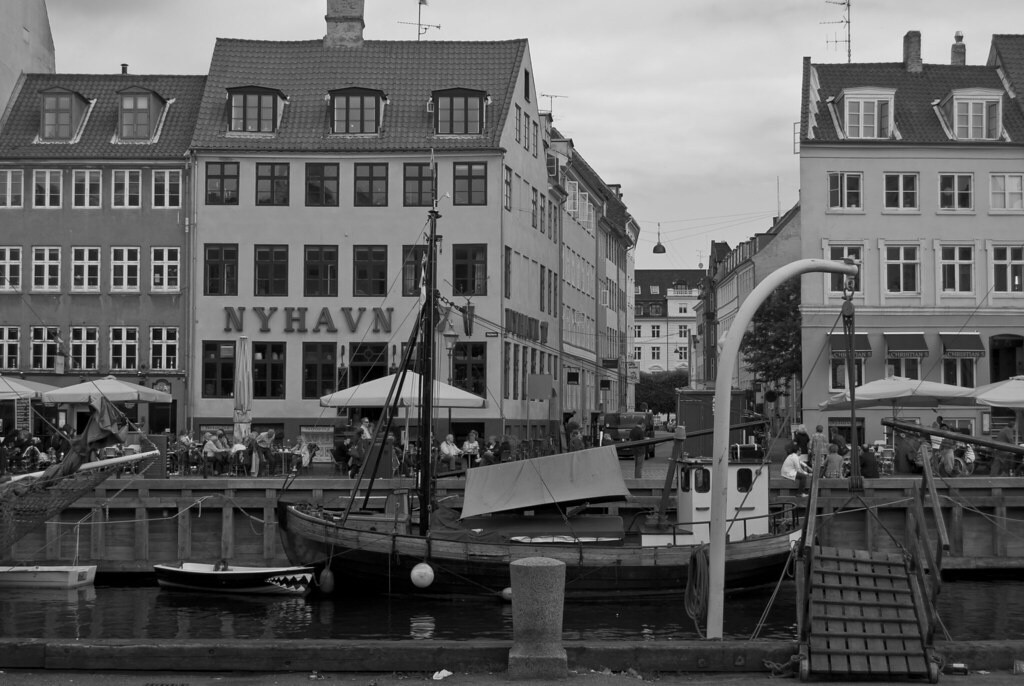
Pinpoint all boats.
[1,563,98,591]
[880,240,1024,577]
[1,274,161,560]
[153,554,315,598]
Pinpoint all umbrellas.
[975,375,1024,444]
[320,368,489,452]
[818,374,976,426]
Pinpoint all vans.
[601,411,656,460]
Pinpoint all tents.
[41,376,172,434]
[0,374,64,436]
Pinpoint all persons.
[0,414,1019,485]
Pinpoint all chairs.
[457,437,546,464]
[845,444,895,474]
[165,440,320,477]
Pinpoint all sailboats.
[274,144,821,607]
